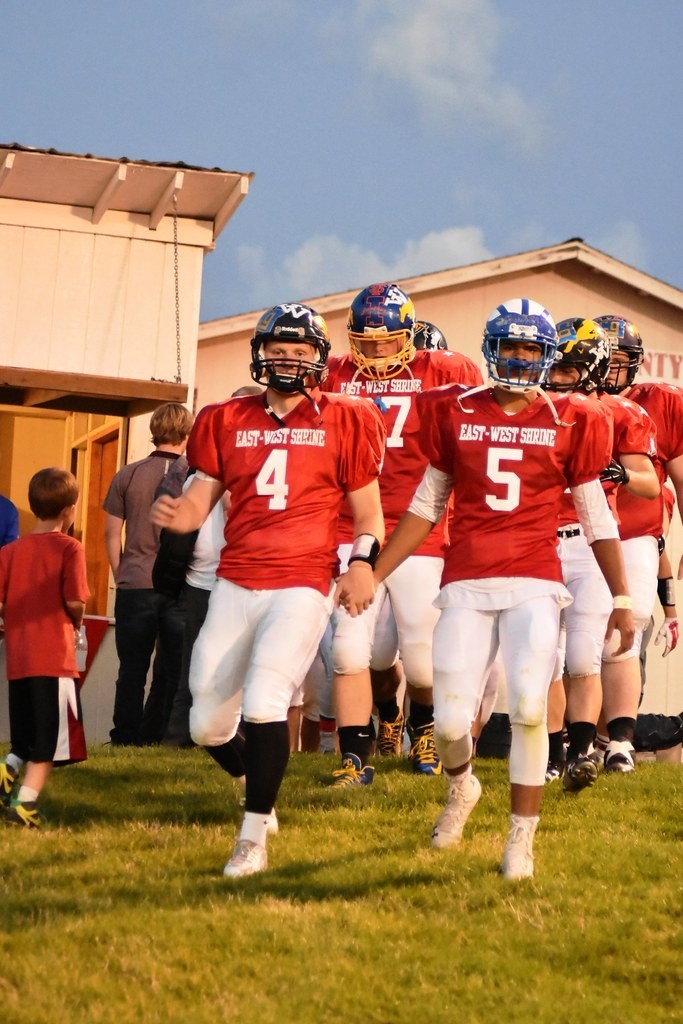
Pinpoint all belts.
[558,529,580,538]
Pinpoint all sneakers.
[503,815,540,880]
[266,806,279,834]
[604,737,636,773]
[9,798,43,830]
[377,706,405,758]
[0,763,20,811]
[328,752,375,787]
[222,839,268,877]
[406,716,442,775]
[432,774,482,847]
[546,763,564,783]
[563,740,597,792]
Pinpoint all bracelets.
[611,594,634,611]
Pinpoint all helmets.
[593,315,644,394]
[414,320,448,350]
[542,317,612,391]
[347,283,416,380]
[482,298,559,387]
[250,303,331,393]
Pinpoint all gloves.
[599,459,629,485]
[654,617,680,657]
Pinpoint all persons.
[101,280,683,881]
[0,467,90,829]
